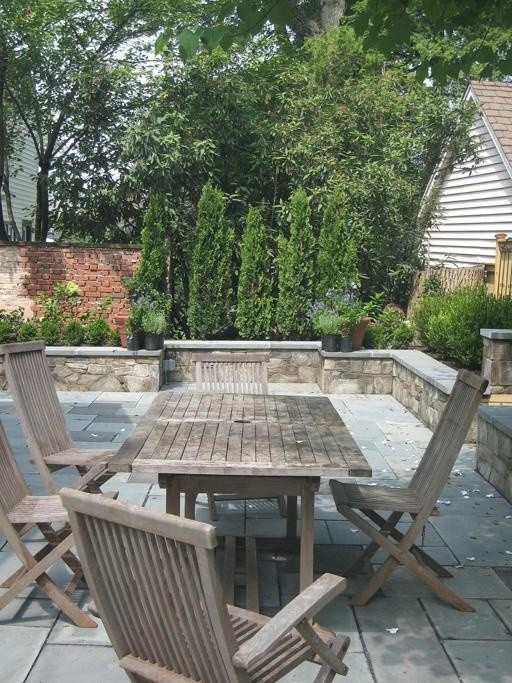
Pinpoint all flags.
[128,332,165,350]
[320,320,369,351]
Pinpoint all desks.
[106,392,372,625]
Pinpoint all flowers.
[308,275,364,334]
[124,292,169,333]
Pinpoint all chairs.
[2,342,119,495]
[329,368,489,613]
[59,487,351,683]
[192,353,287,521]
[1,427,119,627]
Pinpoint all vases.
[124,292,169,333]
[128,332,165,350]
[308,275,364,334]
[320,320,369,351]
